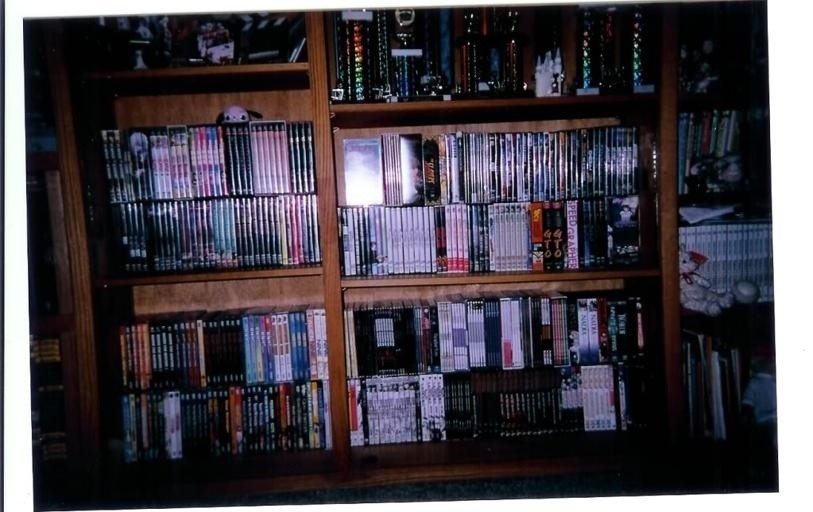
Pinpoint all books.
[27,0,775,473]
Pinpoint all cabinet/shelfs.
[29,13,763,482]
